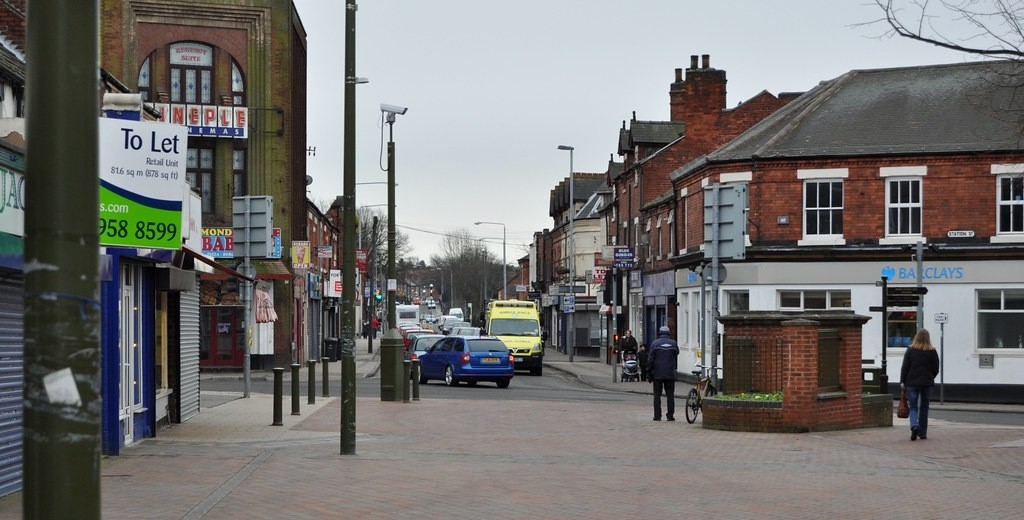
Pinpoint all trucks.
[396,304,419,328]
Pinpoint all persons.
[363,316,378,339]
[647,326,680,421]
[618,330,650,382]
[541,325,547,356]
[900,329,940,441]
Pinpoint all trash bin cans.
[338,338,341,360]
[862,368,883,395]
[324,338,339,362]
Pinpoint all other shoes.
[653,416,675,421]
[910,425,926,441]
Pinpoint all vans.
[413,296,436,309]
[449,308,464,321]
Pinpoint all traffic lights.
[612,335,620,354]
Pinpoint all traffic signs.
[935,313,949,323]
[869,280,928,312]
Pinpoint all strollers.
[620,351,639,383]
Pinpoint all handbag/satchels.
[647,370,653,384]
[897,390,909,418]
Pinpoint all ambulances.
[484,299,547,376]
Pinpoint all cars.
[407,335,446,376]
[420,314,437,323]
[437,315,482,336]
[398,323,435,349]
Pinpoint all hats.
[660,326,669,331]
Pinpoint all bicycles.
[685,364,722,424]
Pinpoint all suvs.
[417,336,514,388]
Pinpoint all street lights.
[357,205,396,337]
[558,145,574,362]
[475,222,506,300]
[430,268,444,294]
[340,77,369,455]
